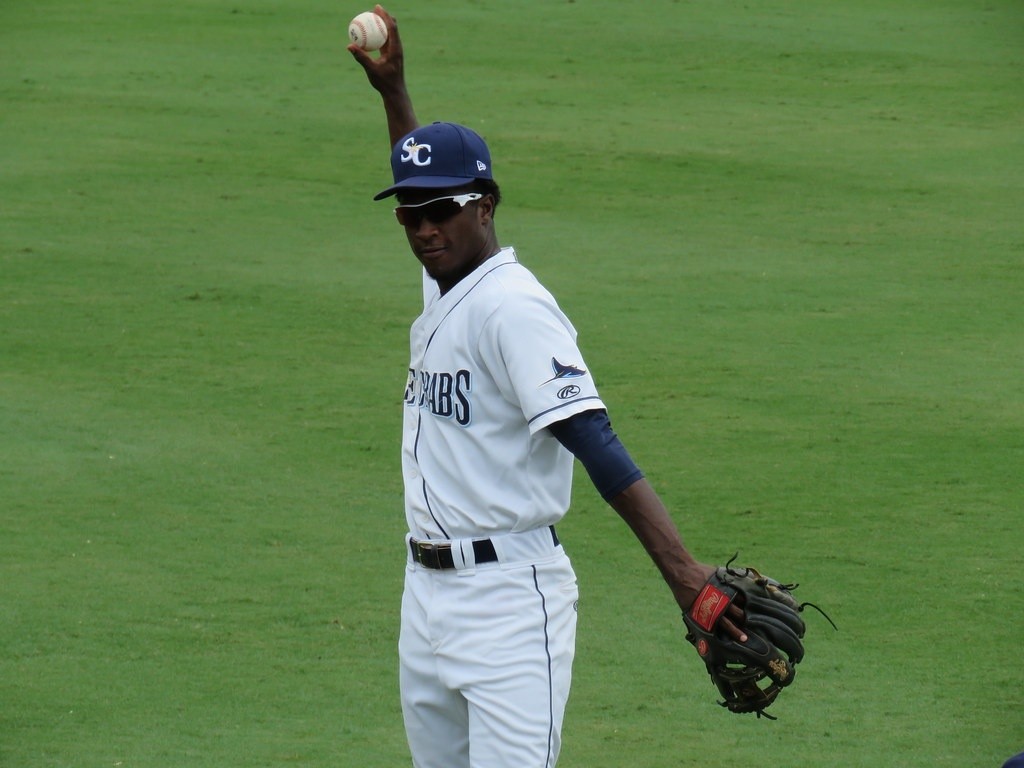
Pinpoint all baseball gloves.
[679,567,807,715]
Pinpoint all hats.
[373,119,492,202]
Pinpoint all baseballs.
[347,11,388,52]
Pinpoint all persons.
[346,4,774,768]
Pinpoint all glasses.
[394,192,484,228]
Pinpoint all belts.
[408,524,561,568]
